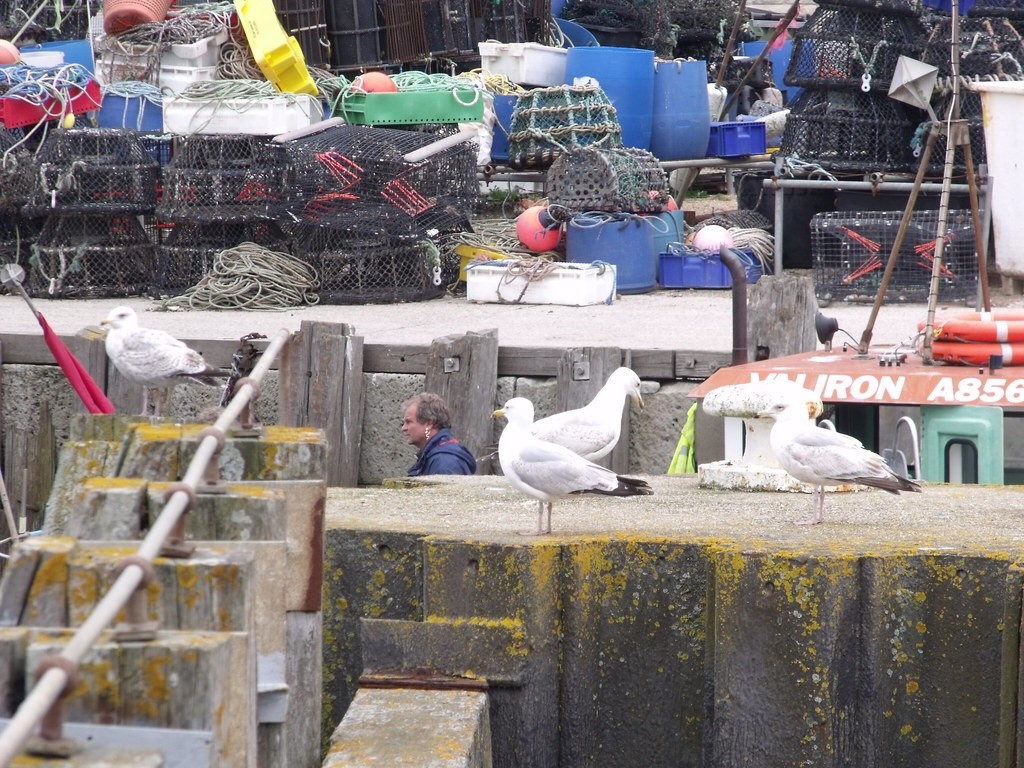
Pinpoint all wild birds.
[99,304,243,418]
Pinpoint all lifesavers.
[917,311,1024,367]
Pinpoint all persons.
[402,393,476,475]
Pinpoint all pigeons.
[489,366,654,536]
[752,395,923,525]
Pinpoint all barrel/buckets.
[736,39,817,104]
[100,96,169,167]
[491,94,516,159]
[21,40,96,76]
[565,46,655,152]
[648,61,710,160]
[566,210,684,295]
[548,16,601,49]
